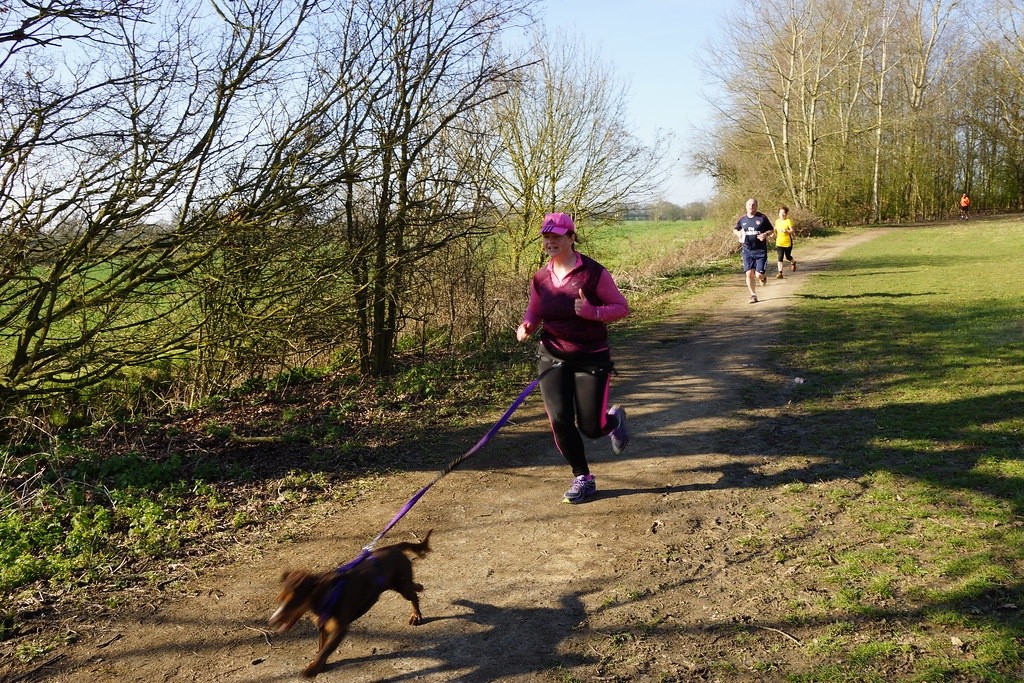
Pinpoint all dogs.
[269,528,435,679]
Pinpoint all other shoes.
[960,217,964,221]
[790,260,796,272]
[776,273,783,278]
[965,218,968,220]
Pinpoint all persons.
[960,193,969,220]
[770,206,796,279]
[735,199,773,303]
[517,213,629,502]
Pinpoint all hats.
[539,212,574,234]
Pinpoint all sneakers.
[608,404,629,455]
[760,276,767,286]
[562,474,596,504]
[749,295,758,302]
